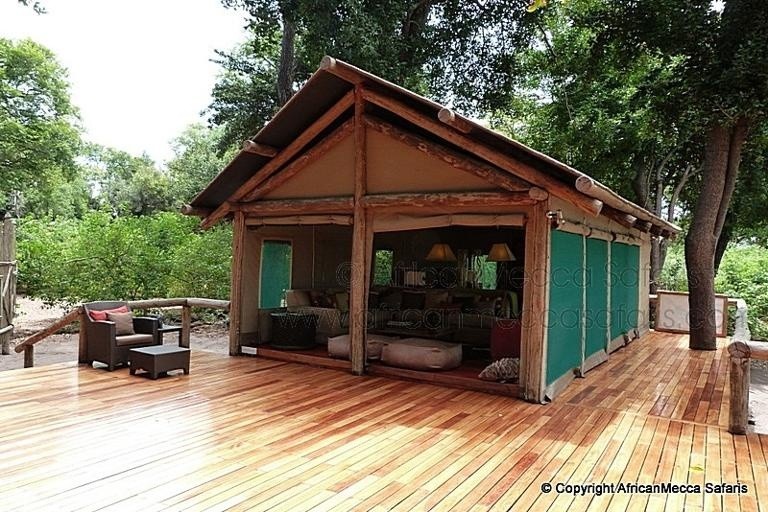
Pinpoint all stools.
[327,334,400,359]
[129,344,191,379]
[382,338,463,370]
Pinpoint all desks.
[269,310,317,351]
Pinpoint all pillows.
[107,311,135,335]
[88,305,128,323]
[478,357,518,382]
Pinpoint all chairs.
[489,318,522,360]
[82,301,159,372]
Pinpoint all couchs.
[287,286,511,343]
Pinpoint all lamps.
[425,243,458,263]
[545,207,565,225]
[485,242,517,263]
